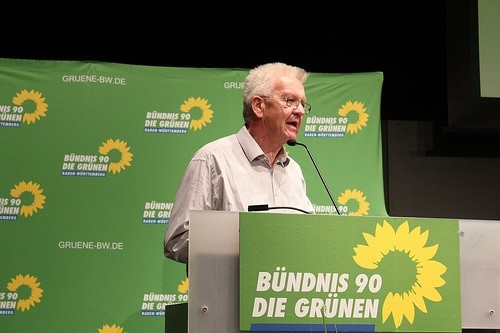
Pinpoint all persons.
[163,62,311,264]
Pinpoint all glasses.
[260,96,312,114]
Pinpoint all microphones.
[248,204,311,214]
[286,139,341,215]
